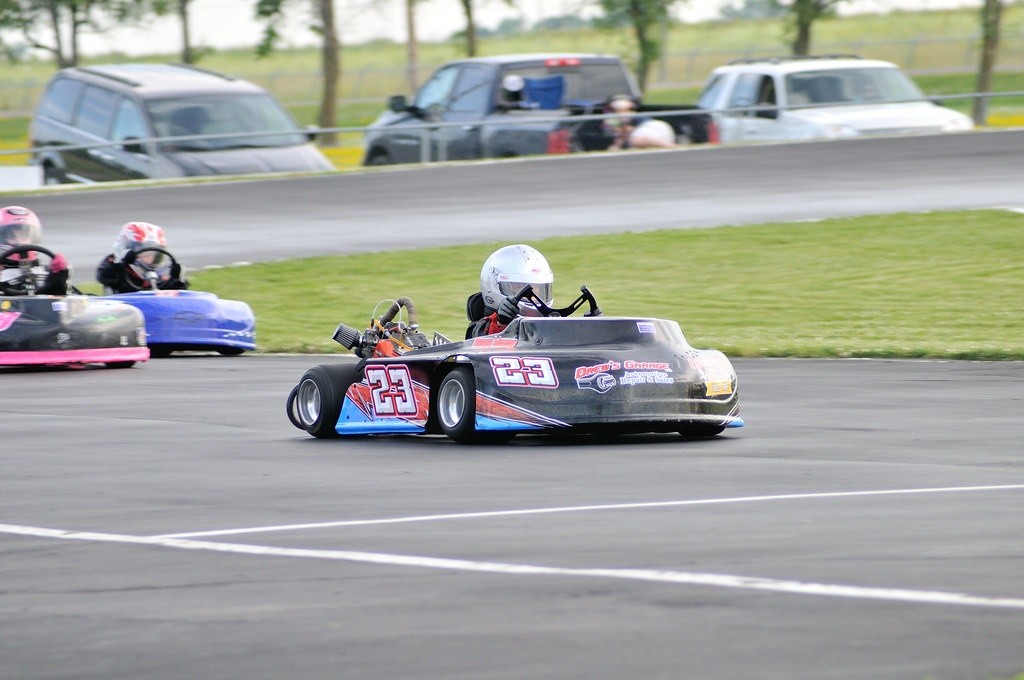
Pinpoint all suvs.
[697,54,975,145]
[26,64,338,186]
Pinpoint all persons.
[464,244,604,340]
[0,206,69,298]
[96,222,190,300]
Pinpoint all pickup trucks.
[363,54,721,171]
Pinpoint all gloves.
[113,245,136,271]
[167,263,182,282]
[497,297,520,324]
[49,255,66,273]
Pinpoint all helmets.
[480,242,555,317]
[114,222,167,272]
[0,206,42,269]
